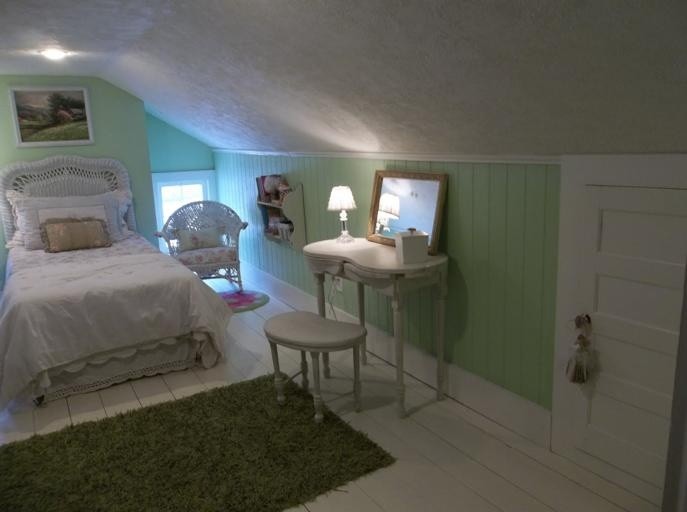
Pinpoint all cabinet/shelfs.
[256,182,307,251]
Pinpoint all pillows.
[13,195,124,251]
[177,225,225,253]
[2,188,135,248]
[38,217,113,253]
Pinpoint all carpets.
[216,289,269,313]
[0,372,398,512]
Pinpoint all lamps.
[326,185,357,243]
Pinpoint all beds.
[0,155,235,414]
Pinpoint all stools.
[263,310,367,425]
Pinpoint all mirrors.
[366,170,448,257]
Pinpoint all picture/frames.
[9,87,94,147]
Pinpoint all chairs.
[163,201,244,292]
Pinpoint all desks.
[302,236,448,419]
